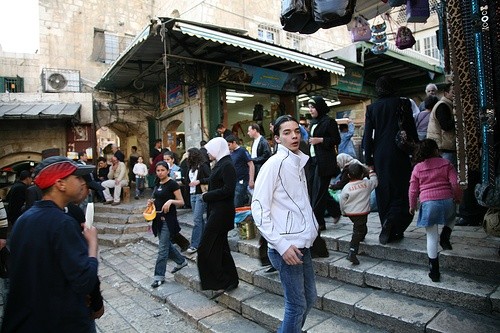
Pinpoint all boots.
[439,226,454,251]
[427,251,442,282]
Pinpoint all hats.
[78,161,106,190]
[32,155,97,190]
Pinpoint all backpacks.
[482,208,500,237]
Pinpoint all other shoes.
[104,200,113,205]
[264,265,276,272]
[346,251,360,265]
[187,247,197,253]
[209,289,224,299]
[320,224,326,230]
[171,261,188,274]
[112,202,121,206]
[135,196,139,200]
[151,280,166,288]
[379,221,404,244]
[332,216,340,223]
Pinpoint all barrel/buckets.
[235,206,255,240]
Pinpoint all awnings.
[347,0,409,32]
[94,17,446,108]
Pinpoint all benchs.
[93,186,130,204]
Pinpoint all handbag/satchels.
[474,183,495,207]
[395,97,417,154]
[280,0,430,55]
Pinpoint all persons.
[0,74,500,333]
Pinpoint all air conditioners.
[42,68,80,93]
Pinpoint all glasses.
[427,90,435,93]
[273,114,293,126]
[163,158,171,161]
